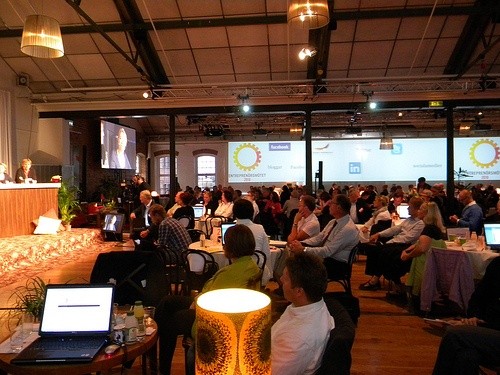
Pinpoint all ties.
[317,220,338,247]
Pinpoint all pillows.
[32,208,66,235]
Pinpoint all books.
[423,318,457,330]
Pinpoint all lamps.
[20,0,64,58]
[287,0,330,30]
[289,117,302,137]
[195,287,272,375]
[459,122,470,134]
[380,126,393,150]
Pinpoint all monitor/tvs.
[103,212,125,232]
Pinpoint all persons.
[273,194,359,295]
[123,175,500,263]
[121,224,260,375]
[381,201,445,304]
[0,162,16,184]
[431,317,500,375]
[109,128,131,169]
[187,252,335,375]
[15,158,38,183]
[106,215,118,231]
[359,196,426,291]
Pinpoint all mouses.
[104,345,121,354]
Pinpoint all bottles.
[134,301,145,334]
[124,311,137,344]
[200,233,205,246]
[471,232,477,242]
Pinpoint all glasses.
[328,202,338,206]
[408,206,416,209]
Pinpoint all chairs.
[180,248,215,297]
[420,247,475,316]
[326,240,361,294]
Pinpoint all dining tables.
[444,240,500,279]
[0,309,158,375]
[189,240,287,279]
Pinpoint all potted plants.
[58,176,82,231]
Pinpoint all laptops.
[483,223,500,251]
[10,284,116,365]
[396,205,411,221]
[192,206,204,221]
[221,222,236,250]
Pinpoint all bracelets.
[363,226,368,229]
[293,223,298,227]
[304,247,308,254]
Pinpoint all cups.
[112,303,118,314]
[268,236,270,242]
[449,234,467,247]
[23,310,33,333]
[144,307,155,320]
[9,318,23,348]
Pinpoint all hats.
[433,184,444,192]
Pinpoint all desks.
[0,183,61,239]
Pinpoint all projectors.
[203,129,224,138]
[344,126,362,134]
[252,129,273,137]
[470,124,492,133]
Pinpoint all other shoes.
[359,280,382,290]
